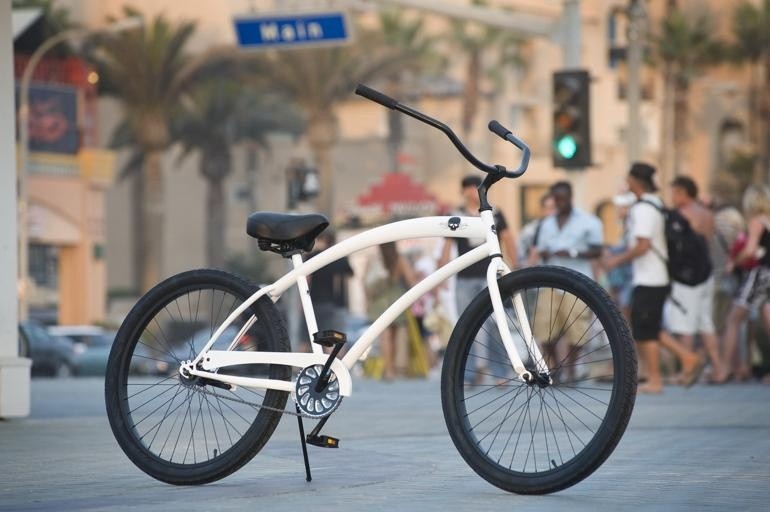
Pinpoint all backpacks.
[638,199,711,286]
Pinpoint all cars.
[603,236,633,294]
[11,320,172,382]
[156,317,383,377]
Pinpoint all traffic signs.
[232,9,355,51]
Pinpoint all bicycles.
[101,83,639,494]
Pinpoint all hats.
[630,163,657,192]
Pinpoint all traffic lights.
[551,69,592,173]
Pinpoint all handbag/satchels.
[730,233,760,268]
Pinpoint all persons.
[229,162,769,393]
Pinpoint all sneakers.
[681,353,708,385]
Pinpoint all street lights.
[19,14,142,325]
